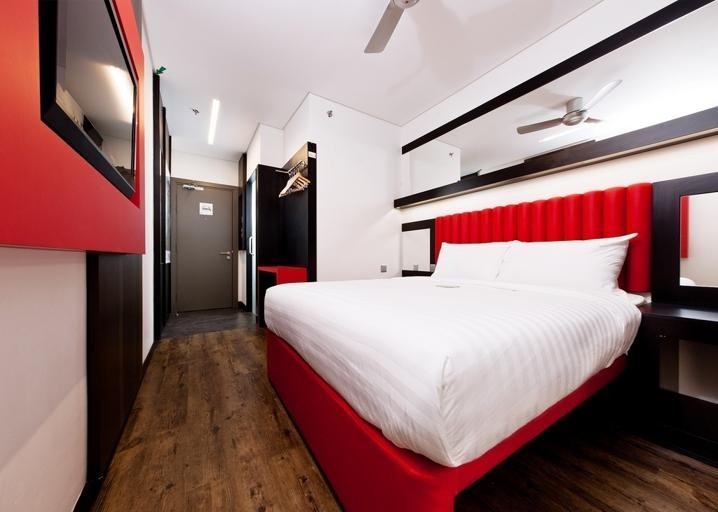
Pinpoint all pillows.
[428,232,638,292]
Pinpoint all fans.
[516,77,623,136]
[363,0,419,55]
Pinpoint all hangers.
[278,162,311,198]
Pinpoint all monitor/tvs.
[38,0,140,198]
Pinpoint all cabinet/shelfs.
[257,265,308,330]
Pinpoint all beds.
[265,179,656,511]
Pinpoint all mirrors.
[402,227,431,272]
[677,190,718,289]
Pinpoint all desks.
[629,302,718,470]
